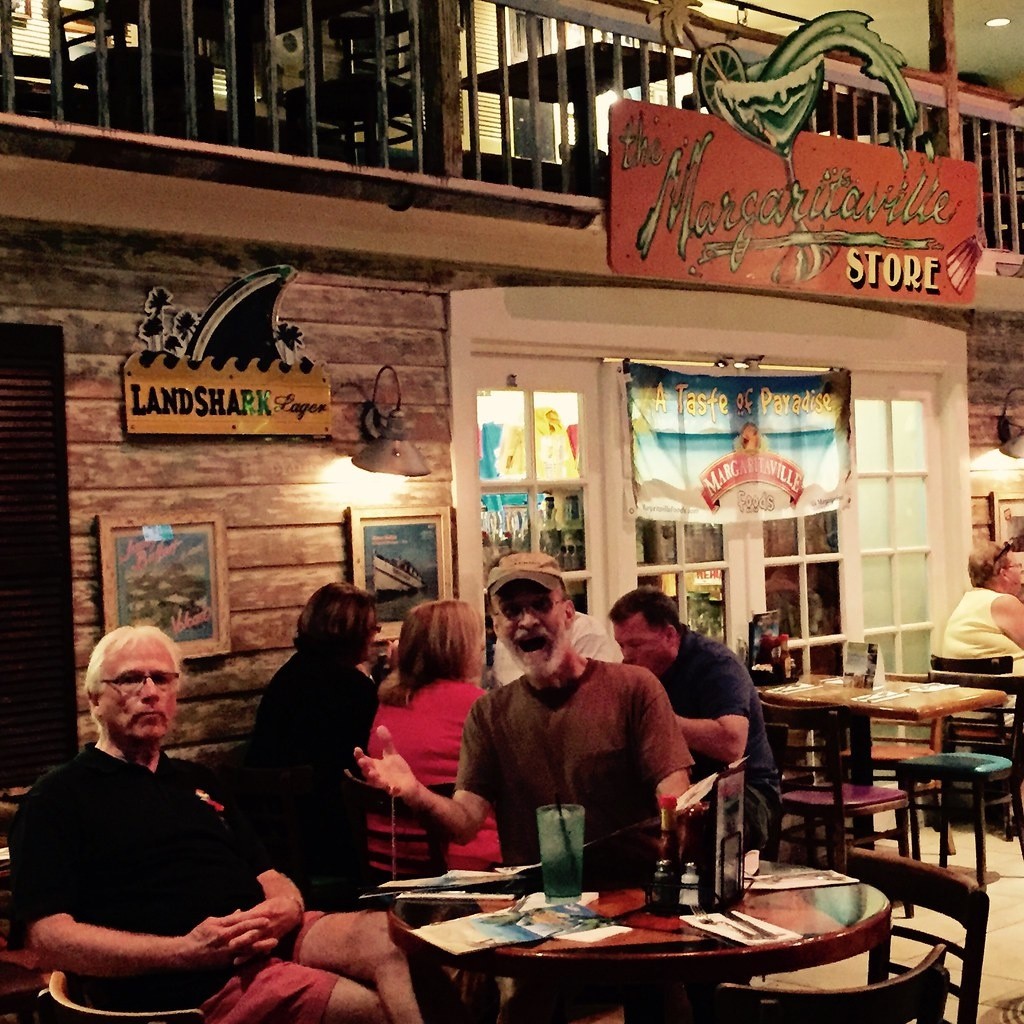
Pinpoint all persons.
[6,626,424,1024]
[607,584,776,867]
[230,581,400,917]
[941,541,1024,739]
[353,551,698,897]
[364,600,505,881]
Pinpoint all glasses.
[996,539,1014,561]
[486,595,566,619]
[996,562,1024,574]
[373,622,382,635]
[100,670,181,693]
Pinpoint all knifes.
[760,873,831,880]
[716,906,777,938]
[867,692,904,704]
[782,687,812,693]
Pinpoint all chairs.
[0,653,1024,1024]
[63,0,425,167]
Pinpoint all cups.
[535,803,586,903]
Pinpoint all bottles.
[791,659,795,675]
[780,634,792,681]
[679,861,700,908]
[679,806,707,864]
[656,795,677,862]
[842,672,854,692]
[651,859,677,916]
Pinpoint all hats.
[487,551,569,596]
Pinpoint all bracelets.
[285,895,304,919]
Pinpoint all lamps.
[350,362,435,477]
[998,387,1024,460]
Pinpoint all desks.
[385,858,892,1023]
[462,41,693,198]
[752,673,1007,849]
[802,89,905,140]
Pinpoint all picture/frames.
[98,511,233,662]
[348,504,453,644]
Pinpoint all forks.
[918,684,941,690]
[766,876,846,886]
[689,904,762,940]
